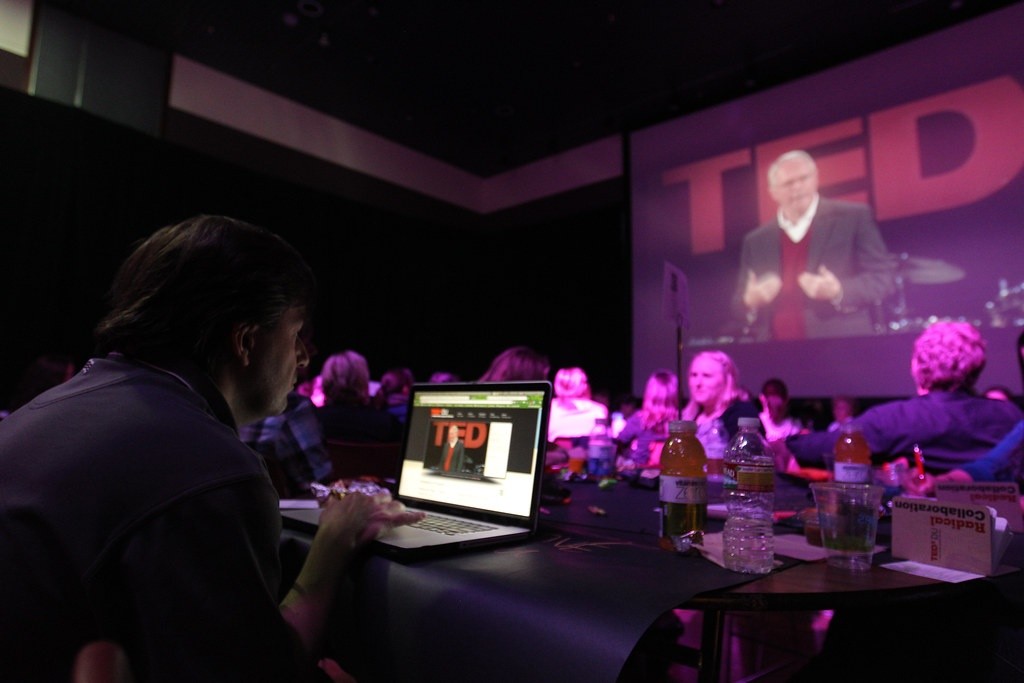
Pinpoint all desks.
[279,481,1024,683]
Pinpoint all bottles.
[587,418,613,479]
[832,425,873,484]
[659,420,707,553]
[704,420,728,482]
[722,417,775,575]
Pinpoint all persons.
[758,378,799,441]
[732,150,893,342]
[439,426,463,472]
[935,329,1024,483]
[682,350,766,458]
[828,400,854,432]
[774,318,1024,466]
[0,351,75,420]
[239,349,460,496]
[480,347,679,475]
[0,214,427,683]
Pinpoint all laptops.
[279,380,553,560]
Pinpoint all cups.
[808,482,886,572]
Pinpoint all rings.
[330,490,341,500]
